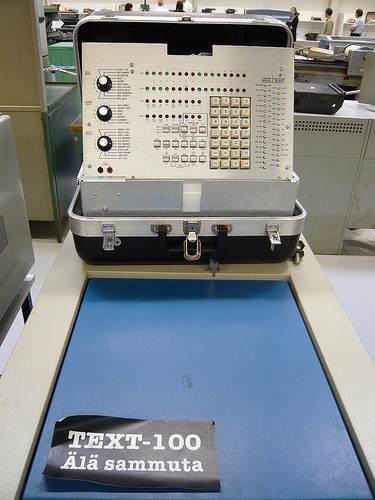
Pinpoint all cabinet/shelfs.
[0,0,82,244]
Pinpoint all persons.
[125,3,133,11]
[285,7,300,48]
[177,0,192,13]
[154,0,169,11]
[350,8,365,37]
[320,7,334,34]
